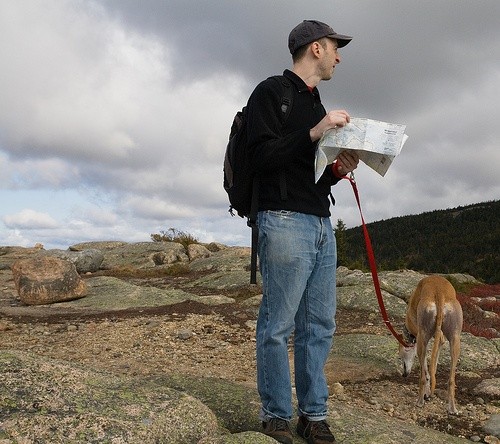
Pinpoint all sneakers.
[297,416,337,444]
[262,418,294,444]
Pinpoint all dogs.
[398,276,463,415]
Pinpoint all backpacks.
[223,75,335,216]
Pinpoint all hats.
[288,19,352,54]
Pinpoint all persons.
[242,20,359,444]
